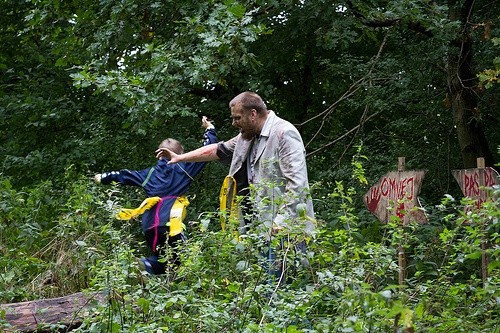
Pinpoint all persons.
[154,92,317,333]
[92,114,219,275]
